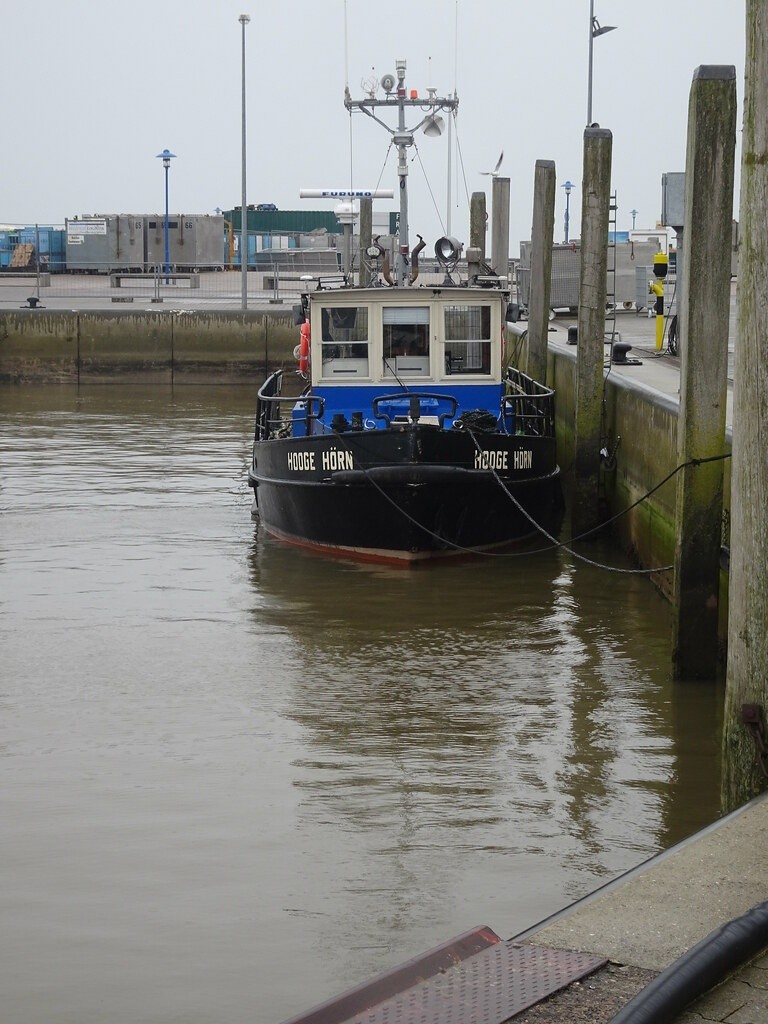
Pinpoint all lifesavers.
[299,322,311,373]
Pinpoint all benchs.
[262,275,354,289]
[110,272,200,288]
[0,272,50,287]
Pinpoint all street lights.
[630,208,639,231]
[155,148,177,287]
[238,14,253,309]
[560,180,576,244]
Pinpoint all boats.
[246,57,565,569]
